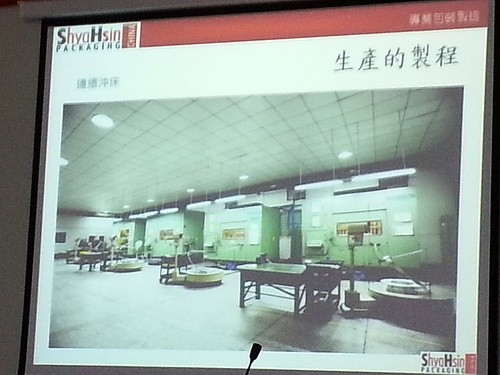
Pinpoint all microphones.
[245,343,262,375]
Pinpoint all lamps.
[111,149,416,225]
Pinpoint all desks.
[236,260,307,318]
[159,250,204,283]
[306,256,346,316]
[78,249,121,269]
